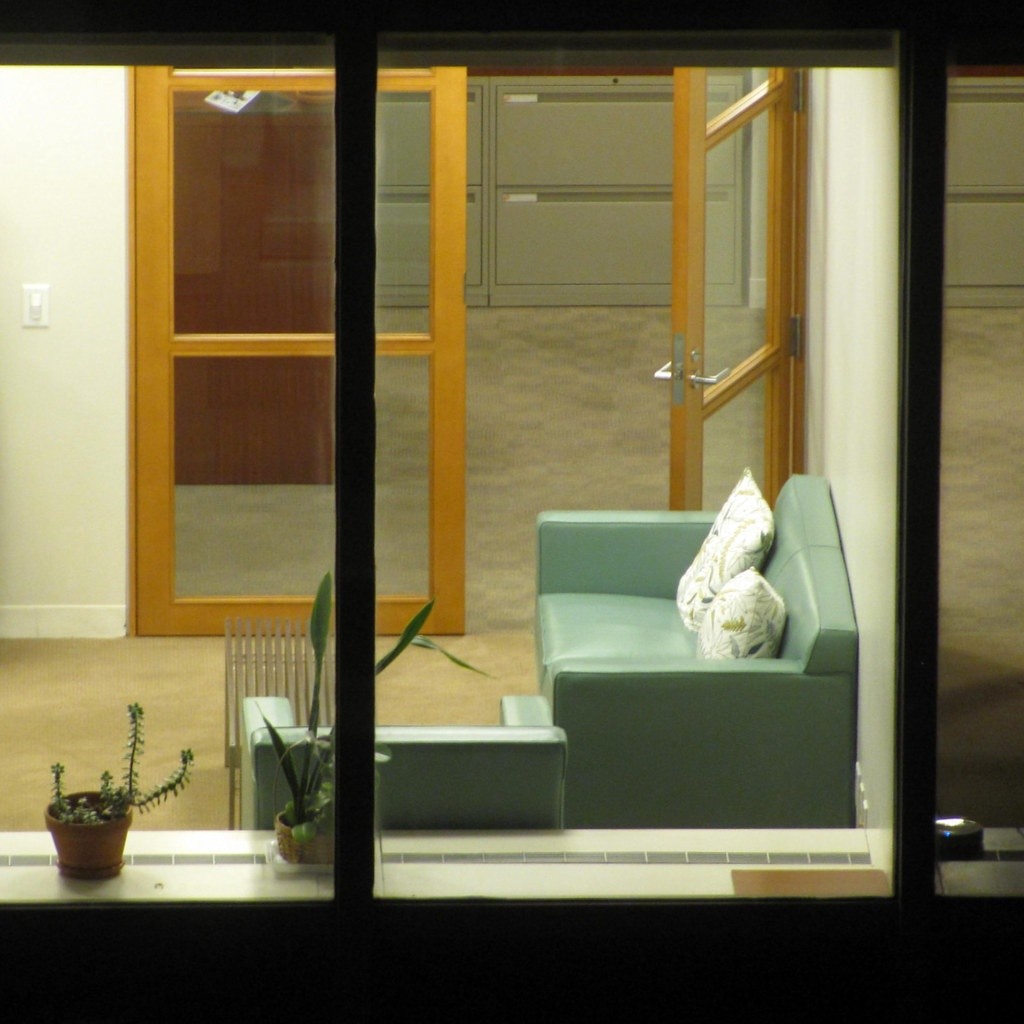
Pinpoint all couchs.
[532,474,861,825]
[237,695,570,831]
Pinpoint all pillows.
[675,467,775,634]
[697,566,788,660]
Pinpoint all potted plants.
[42,702,195,880]
[252,570,499,865]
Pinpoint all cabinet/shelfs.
[173,91,334,486]
[372,72,490,306]
[490,74,745,306]
